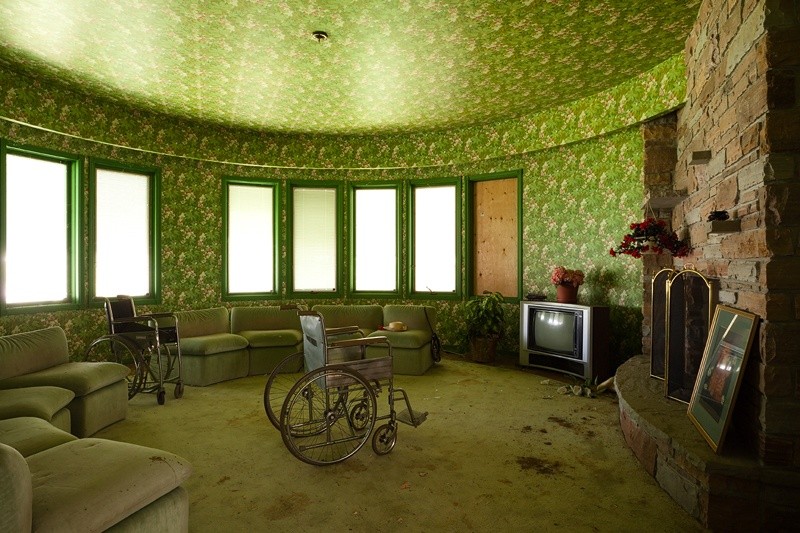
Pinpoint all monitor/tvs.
[519,301,611,384]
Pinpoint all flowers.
[610,217,689,259]
[551,266,585,288]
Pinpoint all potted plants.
[462,291,510,363]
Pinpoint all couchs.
[0,305,437,533]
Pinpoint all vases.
[556,285,579,304]
[634,236,661,254]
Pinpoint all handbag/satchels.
[431,333,442,363]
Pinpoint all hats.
[383,322,408,331]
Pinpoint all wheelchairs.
[84,297,184,405]
[264,310,428,467]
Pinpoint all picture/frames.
[686,304,759,456]
